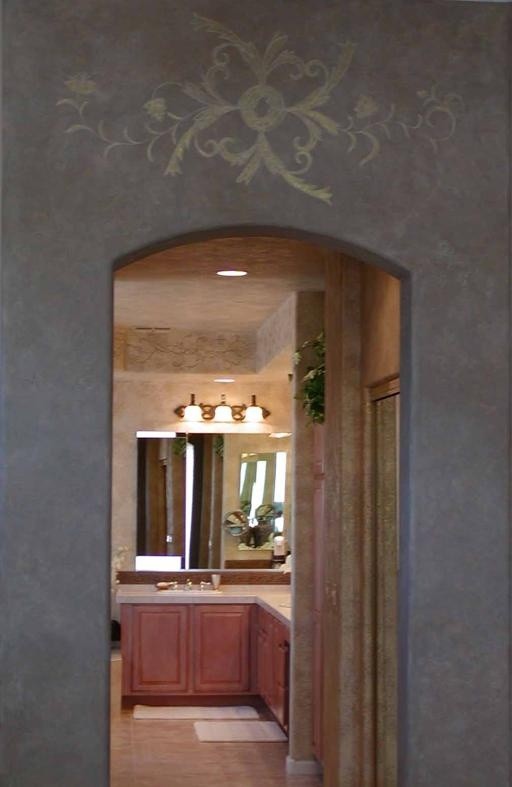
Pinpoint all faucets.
[185,578,193,591]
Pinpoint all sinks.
[157,587,224,595]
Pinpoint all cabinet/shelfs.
[119,597,290,735]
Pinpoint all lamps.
[173,393,271,424]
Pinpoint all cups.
[210,573,222,590]
